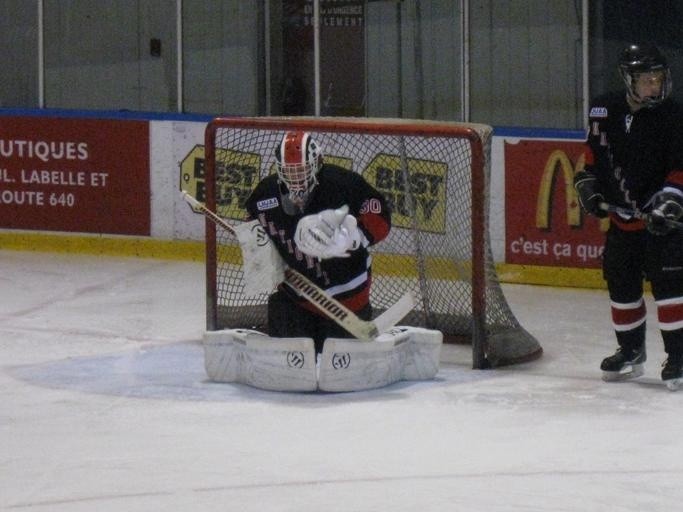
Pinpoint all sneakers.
[601,338,646,371]
[662,346,683,380]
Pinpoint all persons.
[573,39,683,380]
[201,130,445,393]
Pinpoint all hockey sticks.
[180,190,415,343]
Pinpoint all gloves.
[644,182,682,235]
[573,164,606,219]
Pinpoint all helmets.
[274,130,324,205]
[617,38,672,108]
